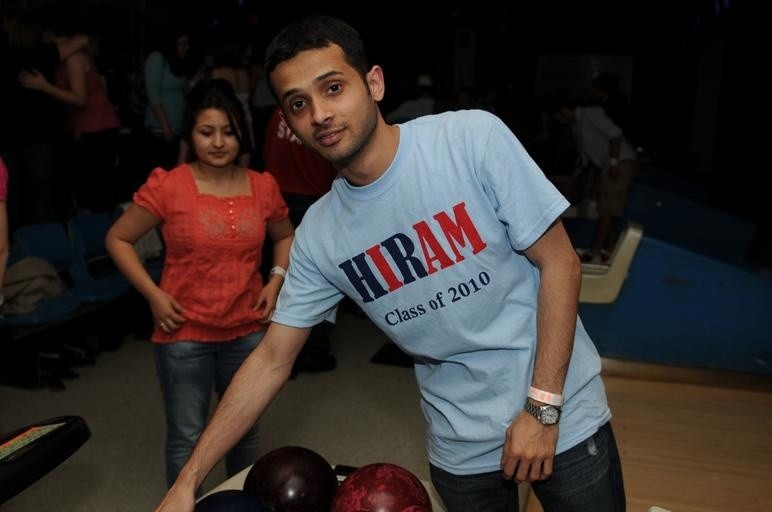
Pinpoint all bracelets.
[609,158,618,167]
[527,385,565,407]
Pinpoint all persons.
[556,92,637,256]
[104,79,295,502]
[154,17,626,512]
[1,33,338,380]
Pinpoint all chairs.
[0,203,167,391]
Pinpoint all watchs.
[523,397,561,425]
